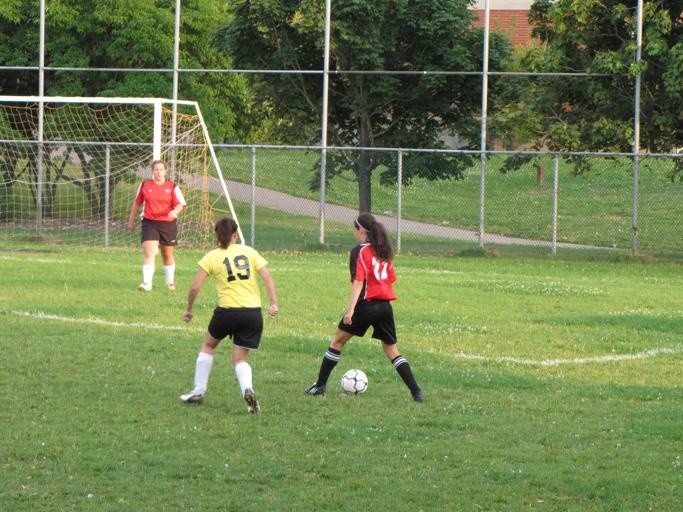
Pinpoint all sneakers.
[139,284,152,290]
[167,282,175,290]
[244,389,260,414]
[413,392,423,401]
[180,393,202,403]
[306,385,323,394]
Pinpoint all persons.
[128,161,187,291]
[306,214,425,402]
[180,218,278,413]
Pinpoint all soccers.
[340,367,369,396]
